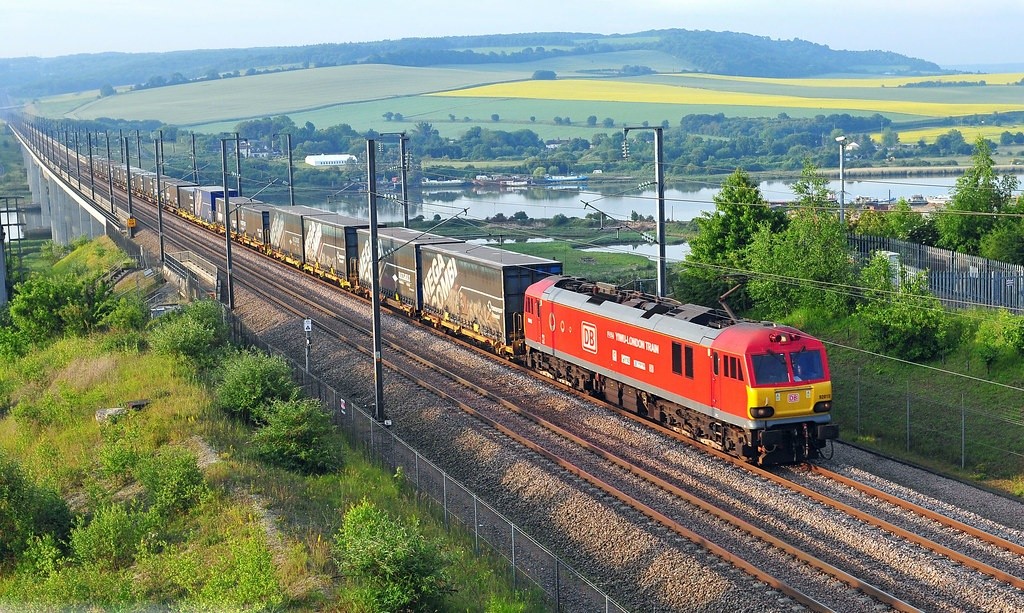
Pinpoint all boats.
[909,198,929,205]
[541,175,588,181]
[496,181,537,186]
[854,195,896,204]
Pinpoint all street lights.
[836,137,847,224]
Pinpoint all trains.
[85,155,833,468]
